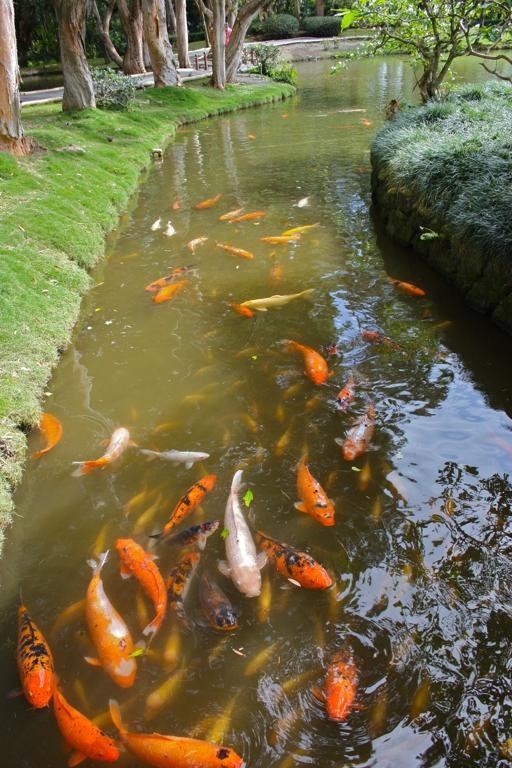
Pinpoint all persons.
[225,19,233,47]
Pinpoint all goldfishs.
[13,328,512,768]
